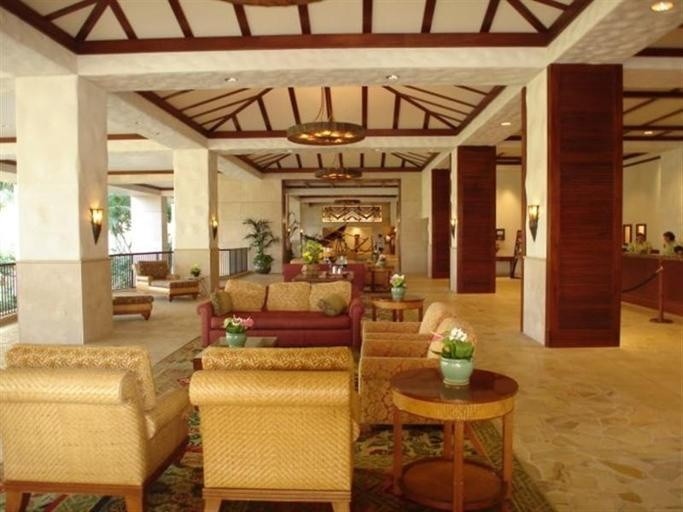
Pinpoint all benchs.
[111,291,155,322]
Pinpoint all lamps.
[89,205,105,247]
[449,217,456,239]
[282,86,367,147]
[211,221,218,241]
[527,203,541,243]
[319,200,383,224]
[312,151,361,184]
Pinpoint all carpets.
[0,334,558,512]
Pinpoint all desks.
[386,362,519,509]
[183,272,211,300]
[369,292,426,323]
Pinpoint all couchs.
[193,282,362,353]
[278,247,399,292]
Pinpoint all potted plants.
[188,264,200,277]
[240,216,281,276]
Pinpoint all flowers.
[215,311,256,334]
[387,271,408,291]
[294,238,325,265]
[425,324,477,363]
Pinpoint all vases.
[389,286,407,303]
[438,355,475,386]
[223,331,247,348]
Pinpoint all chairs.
[356,299,458,350]
[184,342,367,512]
[129,259,201,304]
[354,316,479,439]
[0,340,192,511]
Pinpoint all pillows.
[207,288,233,317]
[315,291,348,318]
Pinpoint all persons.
[374,233,385,253]
[660,231,681,257]
[626,232,651,255]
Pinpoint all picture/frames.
[622,223,632,245]
[634,223,646,242]
